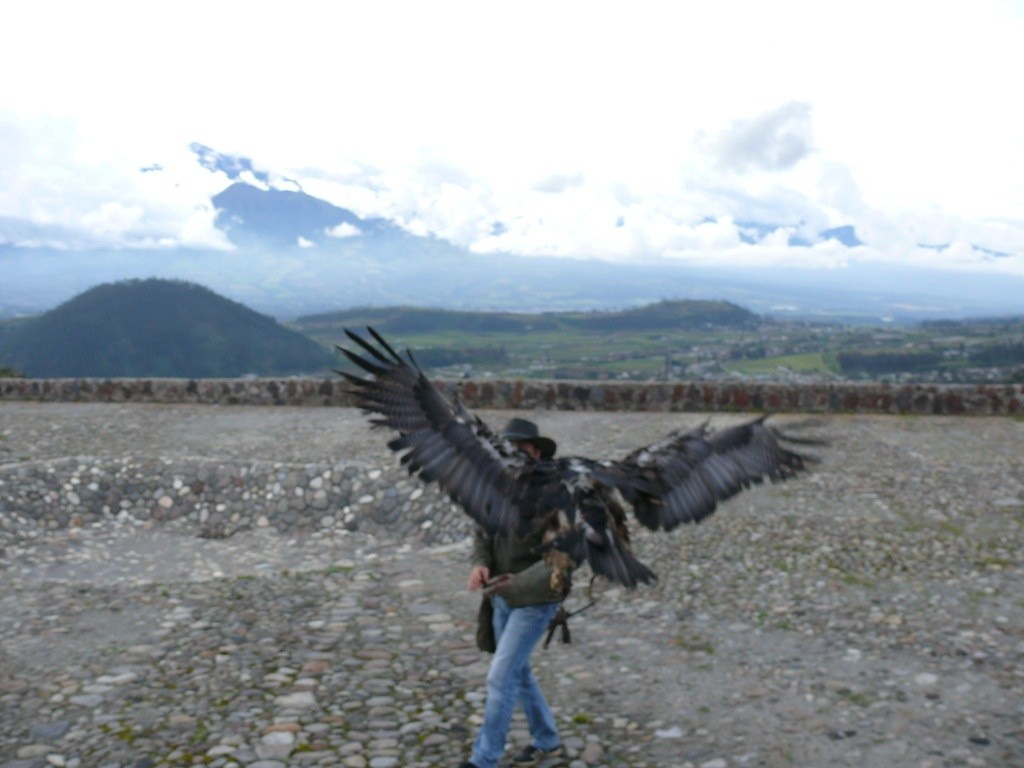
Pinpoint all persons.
[459,417,584,768]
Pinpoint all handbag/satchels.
[476,597,496,654]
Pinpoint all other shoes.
[513,744,567,766]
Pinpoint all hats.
[498,418,557,461]
